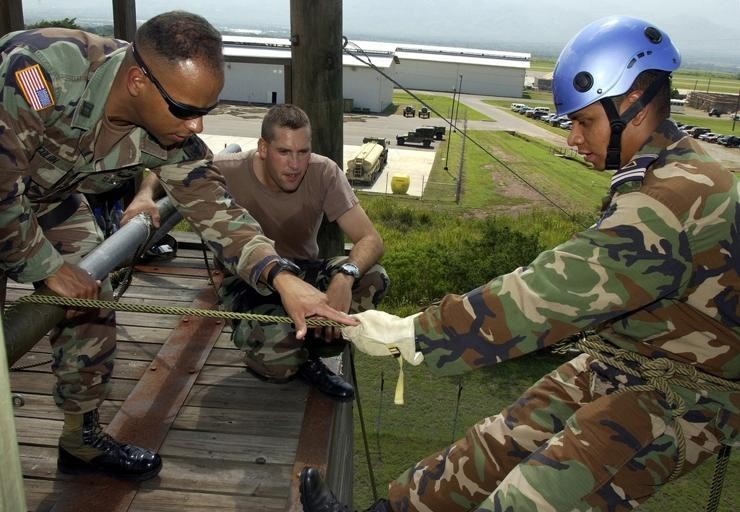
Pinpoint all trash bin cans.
[344,99,353,113]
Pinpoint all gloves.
[340,310,425,367]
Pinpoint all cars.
[511,102,573,130]
[675,108,740,147]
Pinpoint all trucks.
[346,136,390,186]
[395,126,446,148]
[670,90,687,100]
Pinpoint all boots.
[299,466,391,512]
[59,410,163,479]
[305,354,356,402]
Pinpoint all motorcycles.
[403,104,415,117]
[418,106,430,118]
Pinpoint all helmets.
[550,15,679,118]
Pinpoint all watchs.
[338,263,359,279]
[268,257,302,290]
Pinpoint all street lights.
[444,73,463,170]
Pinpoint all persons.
[119,103,390,401]
[1,10,362,481]
[300,16,740,512]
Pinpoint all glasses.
[130,43,220,118]
